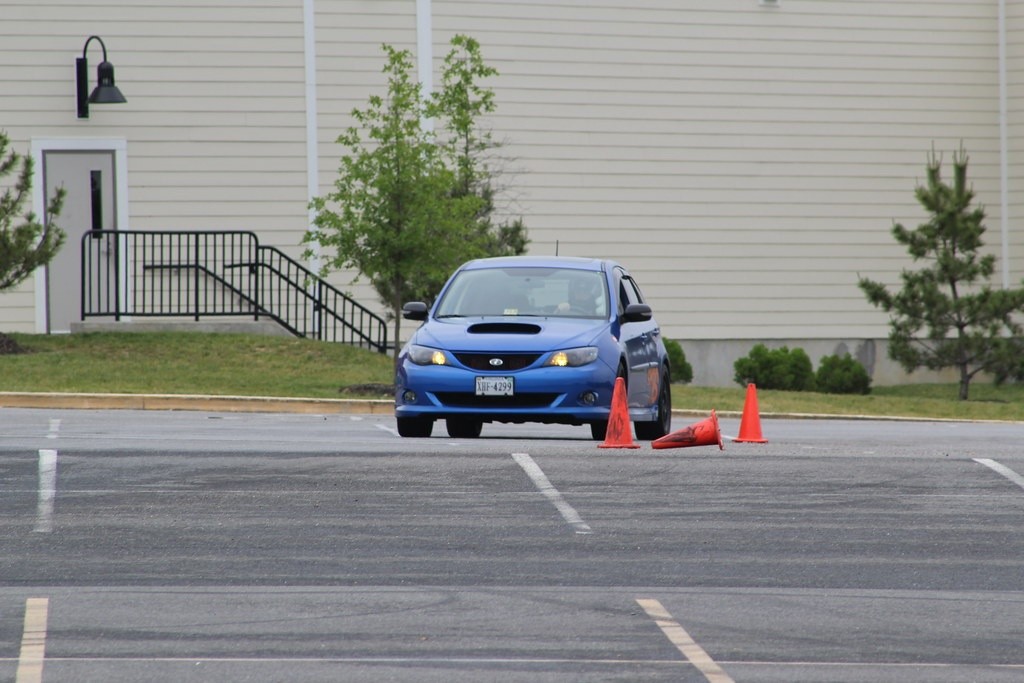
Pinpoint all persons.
[558,274,598,316]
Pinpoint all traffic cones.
[729,381,769,444]
[596,375,641,450]
[650,408,725,451]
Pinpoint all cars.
[392,255,673,441]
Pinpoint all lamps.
[74,36,126,119]
